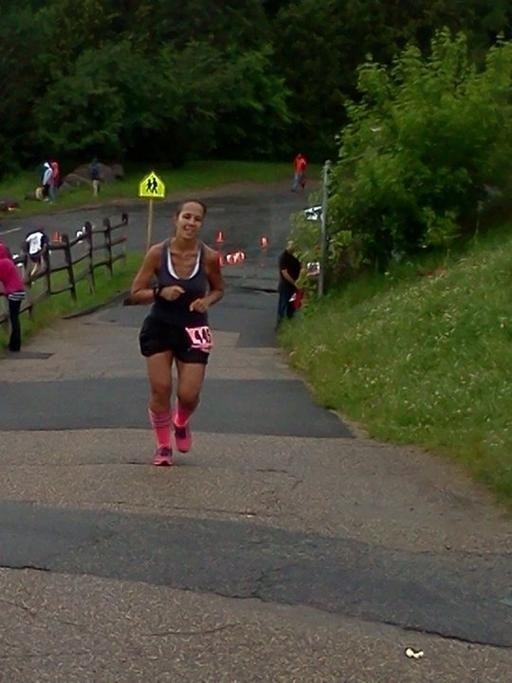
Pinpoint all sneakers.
[170,412,194,453]
[152,443,175,467]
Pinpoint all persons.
[23,223,49,276]
[290,152,308,190]
[276,239,306,330]
[88,155,105,197]
[0,242,25,354]
[128,197,229,467]
[42,161,53,201]
[49,161,60,202]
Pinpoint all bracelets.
[153,285,162,302]
